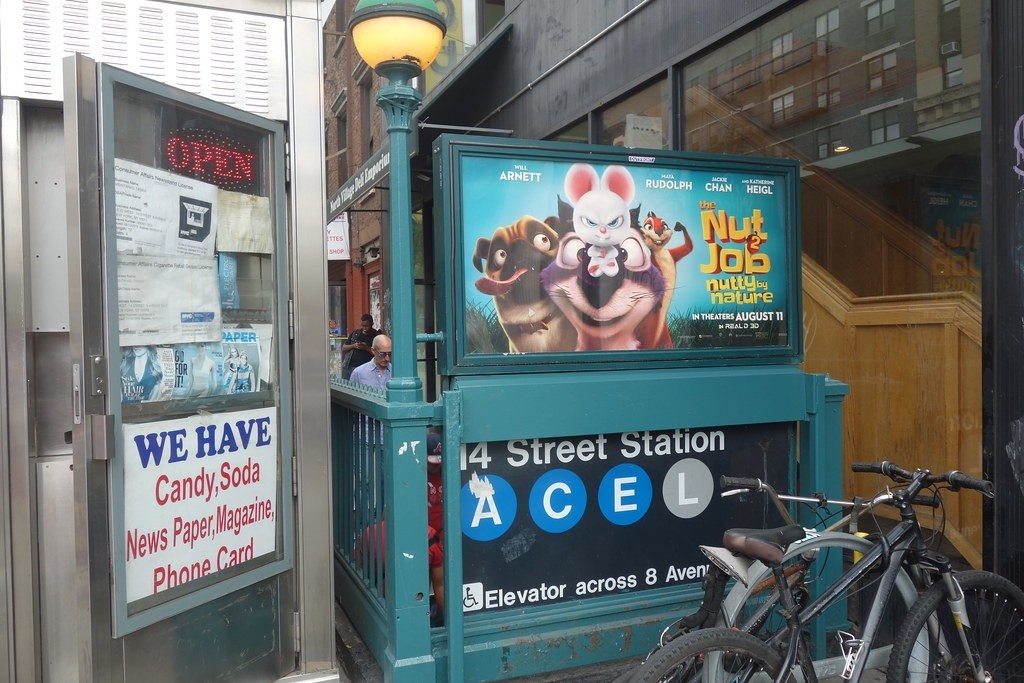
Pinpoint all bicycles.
[610,459,1024,683]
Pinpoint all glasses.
[373,348,392,358]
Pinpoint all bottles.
[337,320,341,336]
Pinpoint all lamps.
[367,247,380,258]
[352,257,367,267]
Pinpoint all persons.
[354,432,446,628]
[342,314,379,380]
[347,335,391,529]
[120,342,256,405]
